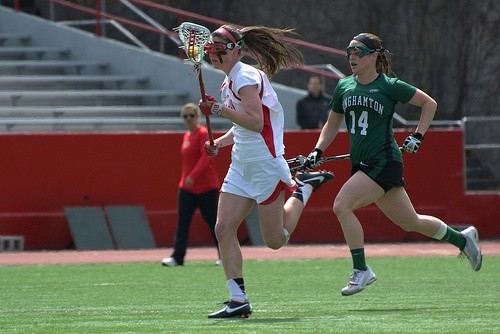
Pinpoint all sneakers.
[340,265,378,296]
[295,171,335,192]
[457,226,483,272]
[207,299,253,318]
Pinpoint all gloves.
[402,132,424,154]
[204,140,220,157]
[299,148,323,174]
[198,93,223,115]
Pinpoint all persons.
[163,103,220,267]
[296,76,332,128]
[199,25,334,318]
[303,32,482,296]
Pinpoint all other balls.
[188,47,198,57]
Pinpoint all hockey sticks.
[178,22,214,145]
[286,147,404,170]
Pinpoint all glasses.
[183,113,195,119]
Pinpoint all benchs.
[0,33,236,132]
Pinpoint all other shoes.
[162,257,178,267]
[215,259,223,266]
